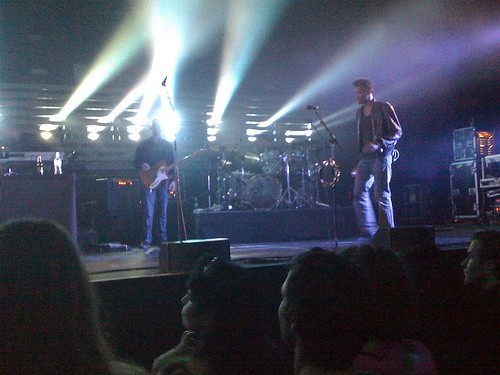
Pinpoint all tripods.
[227,163,257,211]
[273,145,332,209]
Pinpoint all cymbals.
[316,158,342,190]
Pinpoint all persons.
[151,230,500,375]
[134,118,179,248]
[0,217,148,375]
[352,78,403,240]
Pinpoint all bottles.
[36,155,44,176]
[53,152,62,176]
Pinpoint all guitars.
[138,148,209,190]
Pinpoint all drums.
[241,172,282,212]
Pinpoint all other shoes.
[138,240,151,249]
[161,242,168,247]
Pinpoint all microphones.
[305,105,319,110]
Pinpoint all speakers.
[107,178,139,212]
[157,237,230,276]
[373,226,437,253]
